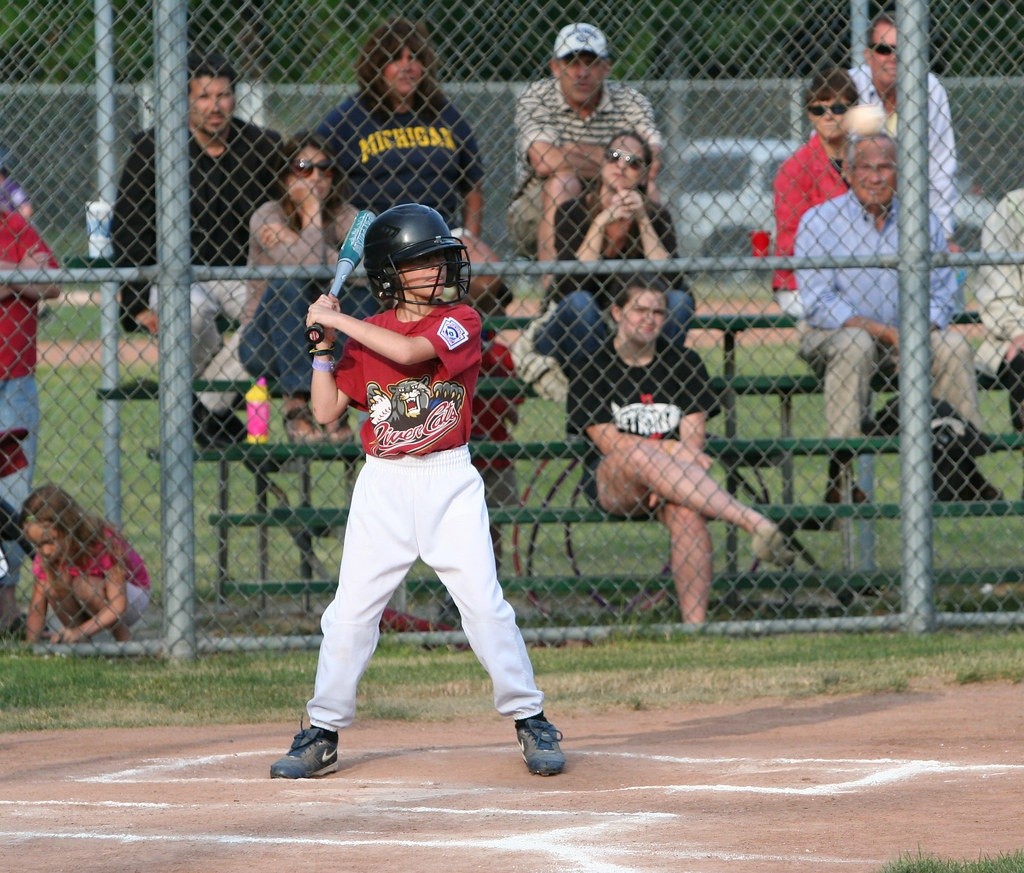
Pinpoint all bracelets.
[311,356,335,371]
[309,341,336,356]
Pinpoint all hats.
[554,24,607,57]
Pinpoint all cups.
[86,202,116,259]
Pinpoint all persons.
[847,11,963,253]
[269,202,565,780]
[566,282,793,622]
[318,18,502,305]
[0,167,32,219]
[534,131,695,379]
[239,131,353,445]
[772,68,860,318]
[508,22,661,287]
[793,135,1001,502]
[20,484,151,643]
[109,53,284,449]
[0,212,61,642]
[973,188,1024,433]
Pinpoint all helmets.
[363,203,472,305]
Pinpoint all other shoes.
[283,406,324,444]
[938,467,1004,501]
[322,414,356,445]
[752,524,794,567]
[0,613,52,641]
[826,480,866,503]
[191,393,248,448]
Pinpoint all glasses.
[603,148,644,170]
[807,102,848,115]
[289,159,334,177]
[868,43,896,55]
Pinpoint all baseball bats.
[304,209,377,344]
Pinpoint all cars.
[656,137,990,286]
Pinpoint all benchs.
[60,253,1023,600]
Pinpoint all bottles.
[245,378,269,443]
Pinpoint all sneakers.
[270,712,339,779]
[517,719,566,775]
